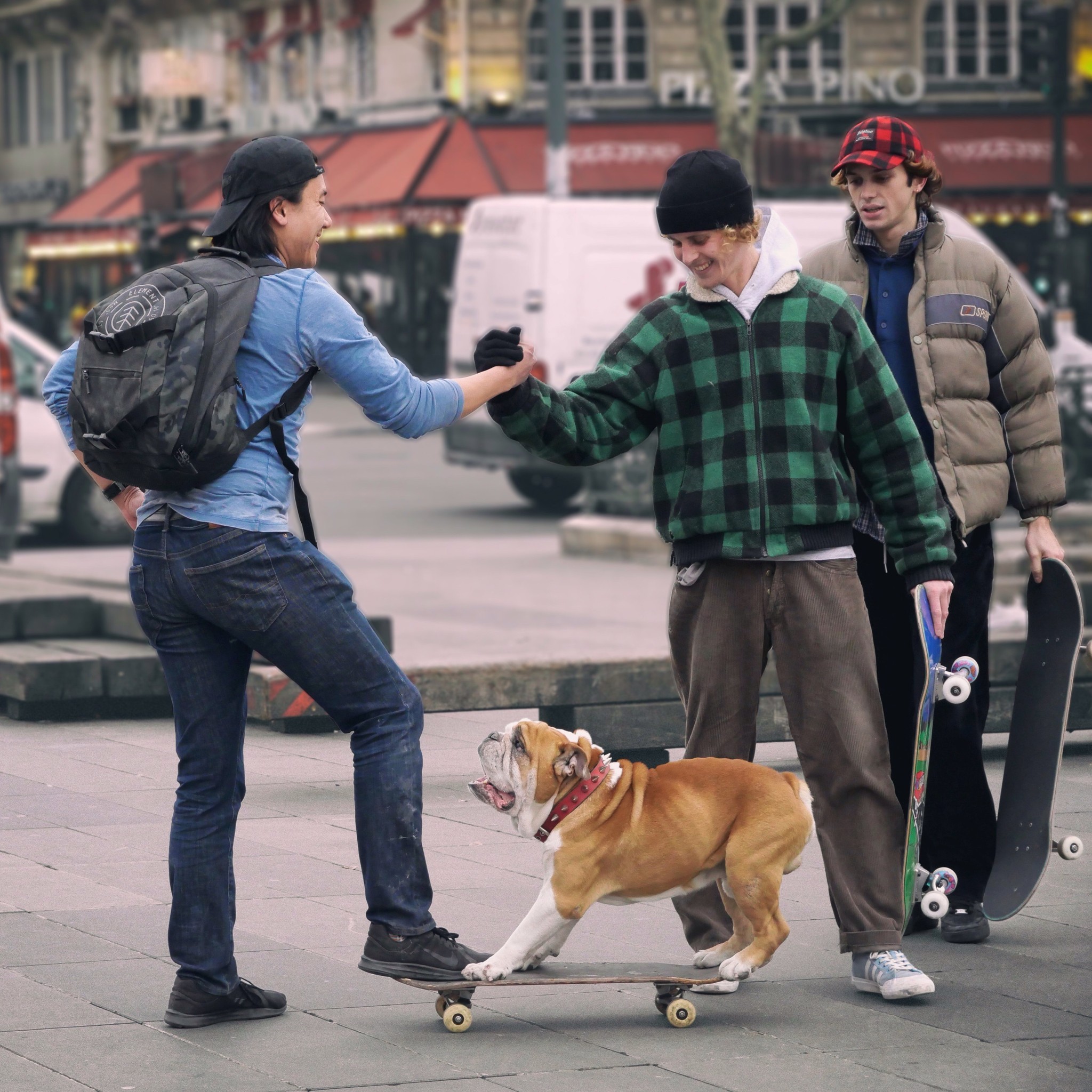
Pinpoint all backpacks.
[68,246,317,493]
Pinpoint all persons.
[39,123,538,1033]
[792,112,1067,942]
[469,140,958,1006]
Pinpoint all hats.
[201,134,325,237]
[829,116,924,177]
[656,150,754,234]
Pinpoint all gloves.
[473,326,524,404]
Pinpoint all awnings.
[20,111,1091,262]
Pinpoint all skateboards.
[980,556,1084,921]
[901,582,978,937]
[385,960,723,1033]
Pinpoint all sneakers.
[164,976,286,1029]
[941,901,990,943]
[358,922,495,981]
[904,902,939,936]
[851,949,935,1000]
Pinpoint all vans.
[441,196,1092,516]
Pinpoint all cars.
[0,310,137,548]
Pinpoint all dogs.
[460,717,814,984]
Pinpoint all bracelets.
[100,479,131,502]
